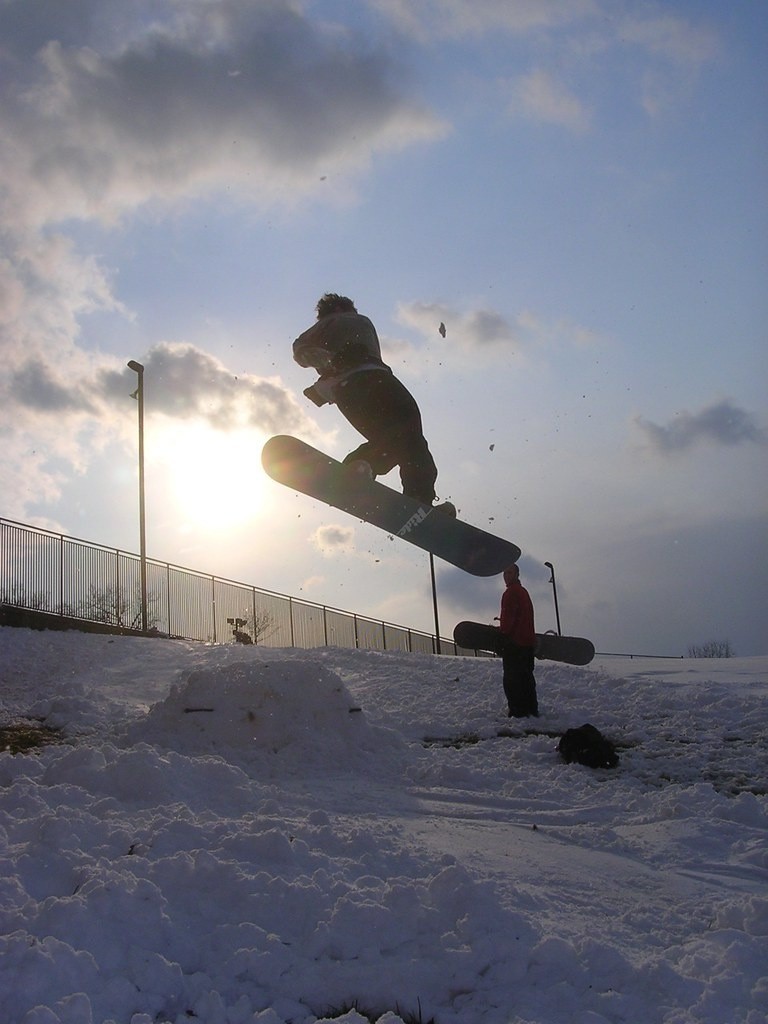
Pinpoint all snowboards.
[453,620,595,666]
[261,435,521,578]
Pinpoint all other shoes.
[403,488,457,519]
[347,459,374,479]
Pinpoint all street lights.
[127,358,146,635]
[544,561,561,637]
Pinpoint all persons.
[496,562,539,718]
[294,295,458,514]
